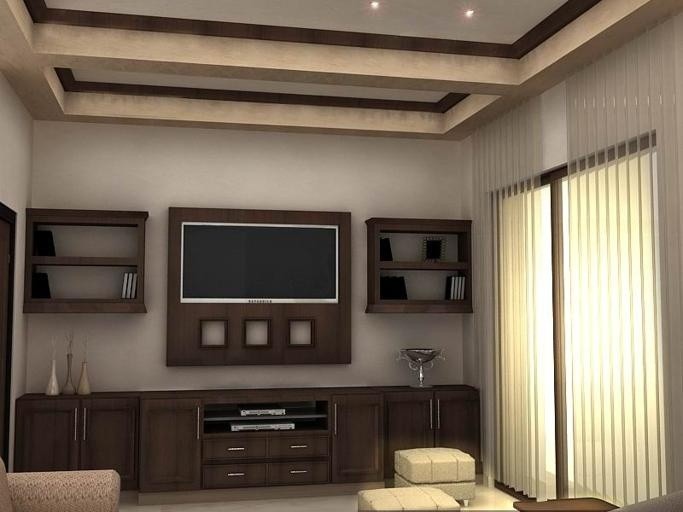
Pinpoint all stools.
[393,445,476,506]
[355,486,461,512]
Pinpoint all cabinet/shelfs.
[23,206,148,316]
[328,386,383,484]
[141,390,203,491]
[199,388,331,488]
[363,216,474,316]
[385,385,483,481]
[13,391,140,493]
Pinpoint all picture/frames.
[420,235,448,265]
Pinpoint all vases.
[75,360,91,396]
[44,357,60,396]
[60,353,75,396]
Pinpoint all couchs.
[0,454,121,512]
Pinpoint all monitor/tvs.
[180,221,339,305]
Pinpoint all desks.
[512,494,620,511]
